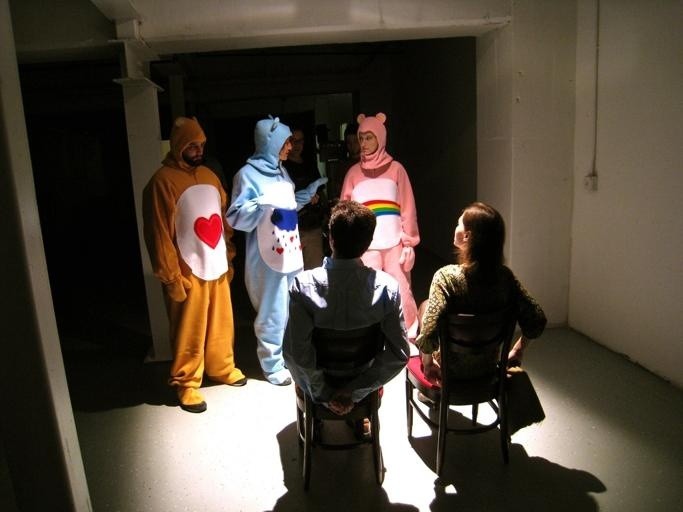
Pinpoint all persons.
[202,122,359,269]
[224,114,332,387]
[414,202,549,411]
[140,115,247,415]
[338,111,423,342]
[280,198,411,442]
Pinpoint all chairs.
[294,306,395,487]
[405,305,517,483]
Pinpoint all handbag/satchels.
[503,362,544,437]
